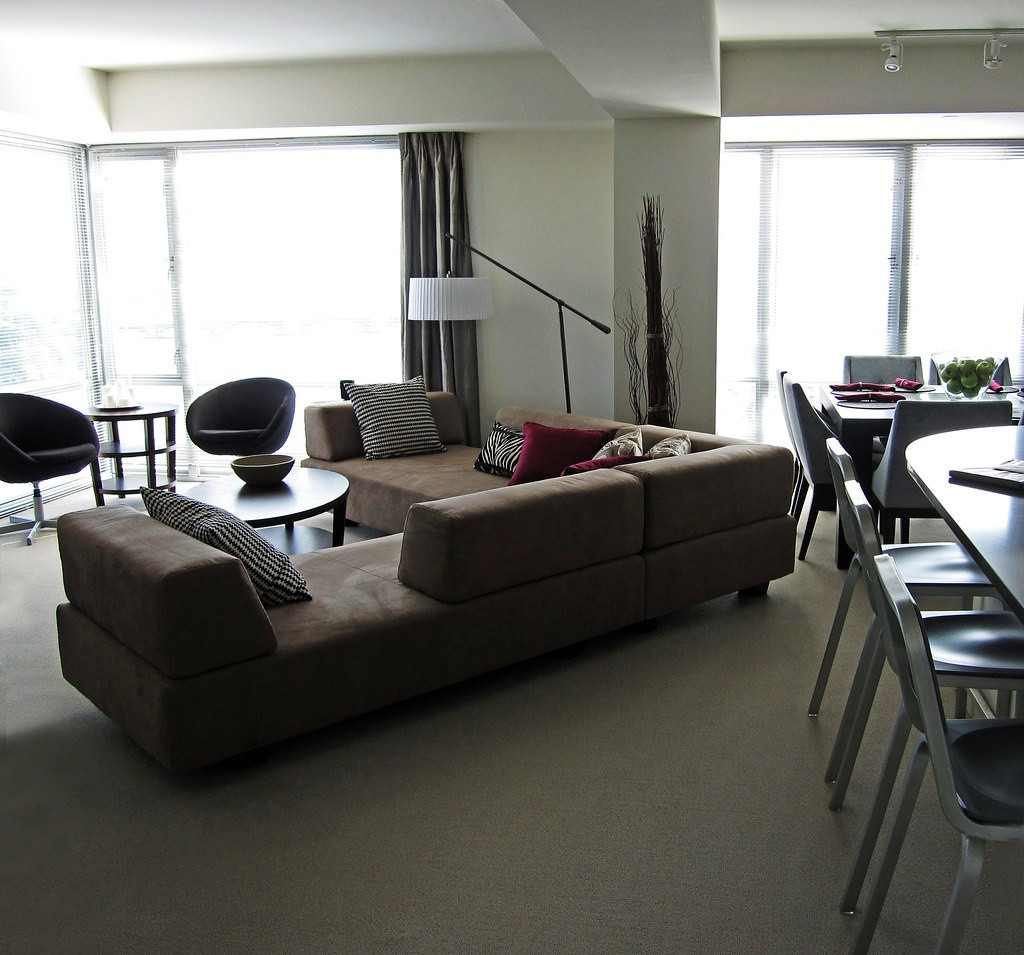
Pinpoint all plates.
[95,403,142,409]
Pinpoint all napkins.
[828,382,896,392]
[894,377,923,390]
[834,393,906,402]
[989,377,1004,393]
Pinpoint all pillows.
[343,375,447,460]
[139,485,313,607]
[508,422,609,487]
[560,455,644,477]
[473,419,524,479]
[644,433,691,460]
[592,427,643,461]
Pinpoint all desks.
[903,425,1024,719]
[184,468,349,548]
[820,385,1024,569]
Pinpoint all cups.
[102,380,137,406]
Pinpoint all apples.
[940,356,995,398]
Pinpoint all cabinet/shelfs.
[83,405,177,507]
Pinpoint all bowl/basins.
[230,454,295,487]
[931,353,1006,401]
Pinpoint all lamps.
[984,34,1007,70]
[408,233,611,414]
[881,34,904,72]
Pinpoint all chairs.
[0,392,101,545]
[186,377,296,456]
[774,356,1024,954]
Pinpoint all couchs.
[55,393,796,777]
